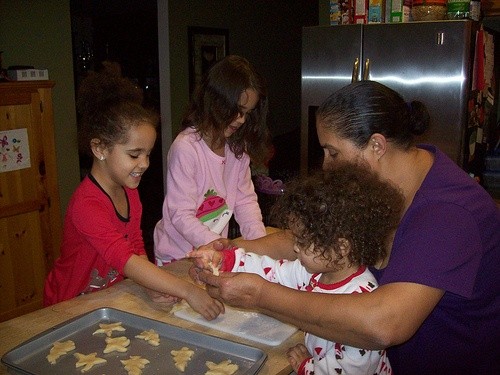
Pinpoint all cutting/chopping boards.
[173,288,299,347]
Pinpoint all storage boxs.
[330,0,411,25]
[483,149,500,188]
[8,69,49,82]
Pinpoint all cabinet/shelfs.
[0,79,63,323]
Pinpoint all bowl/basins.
[411,0,481,24]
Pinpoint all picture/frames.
[186,25,229,102]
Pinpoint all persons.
[44,96,225,321]
[187,81,500,375]
[193,159,402,375]
[154,55,267,267]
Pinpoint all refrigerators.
[296,21,487,185]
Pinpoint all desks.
[1,226,305,375]
